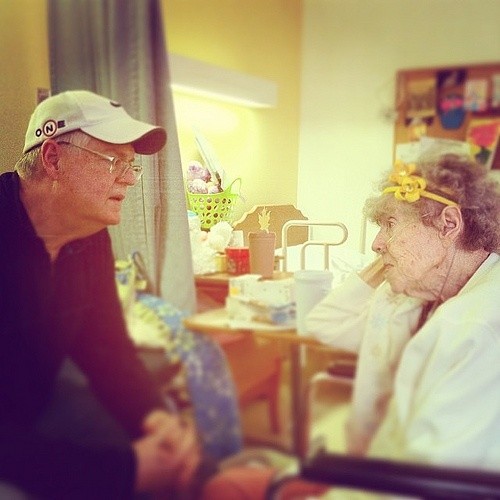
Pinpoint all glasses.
[38,140,143,187]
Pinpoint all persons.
[200,153,500,500]
[0,91,191,500]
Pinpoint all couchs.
[124,291,240,462]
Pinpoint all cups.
[225,246,249,275]
[249,232,276,280]
[294,270,334,337]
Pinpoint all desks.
[186,305,360,472]
[194,272,291,310]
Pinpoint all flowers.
[390,161,426,203]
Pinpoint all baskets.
[185,177,242,230]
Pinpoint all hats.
[23,90,167,155]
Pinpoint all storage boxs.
[224,247,250,275]
